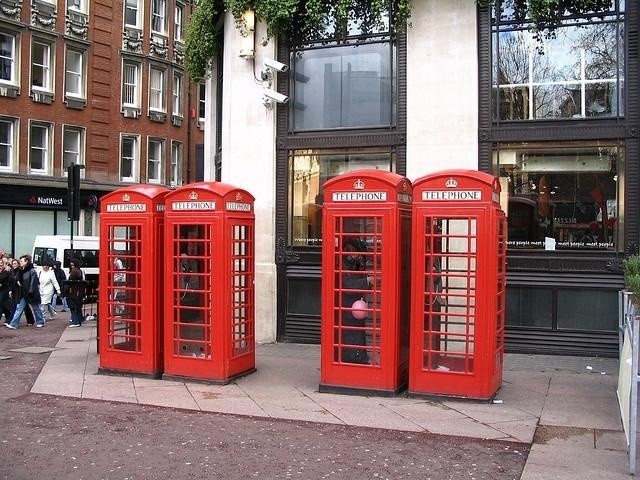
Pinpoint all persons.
[574,200,586,225]
[113,256,124,315]
[423,226,452,370]
[0,248,86,330]
[338,240,374,366]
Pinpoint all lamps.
[238,9,257,61]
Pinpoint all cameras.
[264,56,289,74]
[264,88,290,106]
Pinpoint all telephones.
[180,254,193,287]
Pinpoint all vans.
[31,234,129,303]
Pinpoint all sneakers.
[3,313,80,330]
